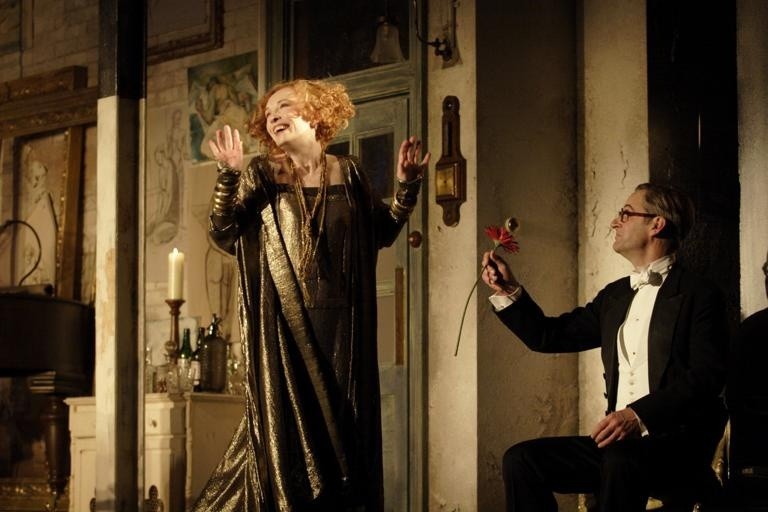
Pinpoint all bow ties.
[630,271,649,291]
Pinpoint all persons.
[188,78,432,512]
[481,183,731,512]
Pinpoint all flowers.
[452,226,519,356]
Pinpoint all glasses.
[619,210,658,223]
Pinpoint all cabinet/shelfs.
[65,393,248,512]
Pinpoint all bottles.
[190,326,207,392]
[200,313,226,392]
[176,328,193,378]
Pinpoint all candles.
[169,247,185,299]
